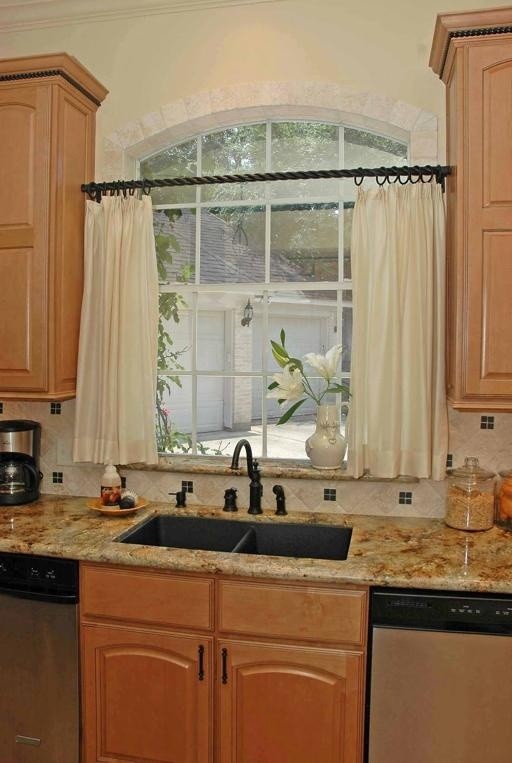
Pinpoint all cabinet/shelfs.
[432,8,512,411]
[0,51,108,403]
[78,562,368,762]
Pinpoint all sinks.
[237,522,354,562]
[110,508,249,553]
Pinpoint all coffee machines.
[0,416,43,505]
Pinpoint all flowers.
[266,326,354,425]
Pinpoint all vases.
[304,401,352,470]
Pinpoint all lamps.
[241,296,254,327]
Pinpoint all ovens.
[0,549,80,762]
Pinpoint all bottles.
[445,454,512,535]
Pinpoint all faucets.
[229,439,266,516]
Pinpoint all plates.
[86,496,150,515]
